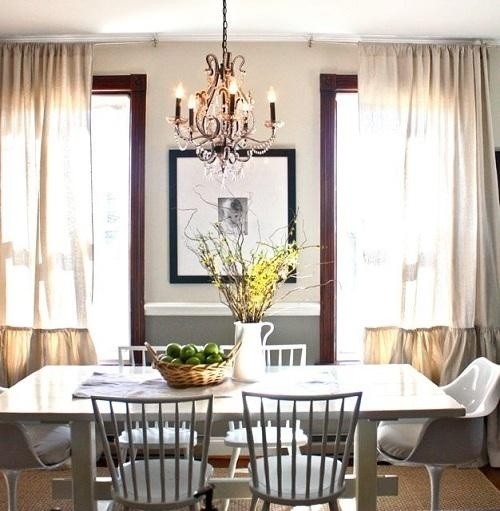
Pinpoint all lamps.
[163,1,286,177]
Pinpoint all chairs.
[116,345,205,484]
[219,343,309,511]
[240,390,363,511]
[89,394,215,511]
[0,386,73,511]
[374,357,500,511]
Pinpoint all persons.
[220,198,248,235]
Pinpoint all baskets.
[142,340,245,389]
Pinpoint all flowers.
[178,179,339,325]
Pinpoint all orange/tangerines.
[158,342,225,365]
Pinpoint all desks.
[1,364,466,511]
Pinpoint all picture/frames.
[168,148,299,285]
